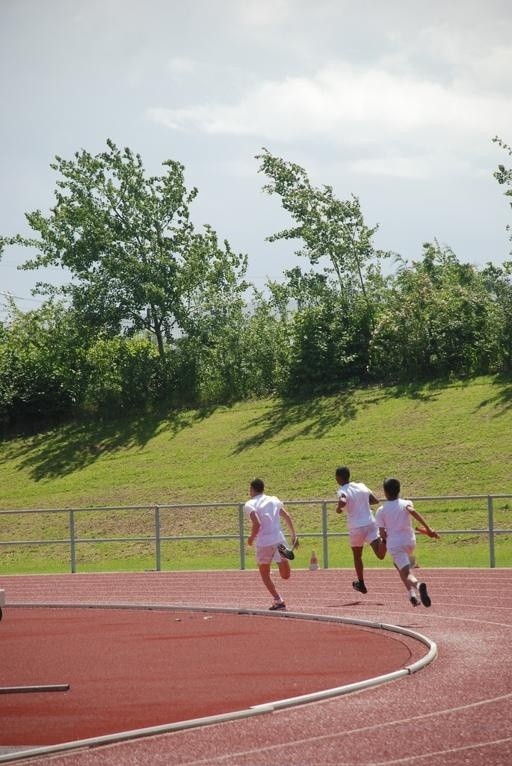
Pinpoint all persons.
[375,477,438,608]
[334,466,386,594]
[243,478,299,611]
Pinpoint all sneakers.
[269,600,286,610]
[418,582,431,607]
[353,581,367,594]
[409,592,420,607]
[278,543,294,560]
